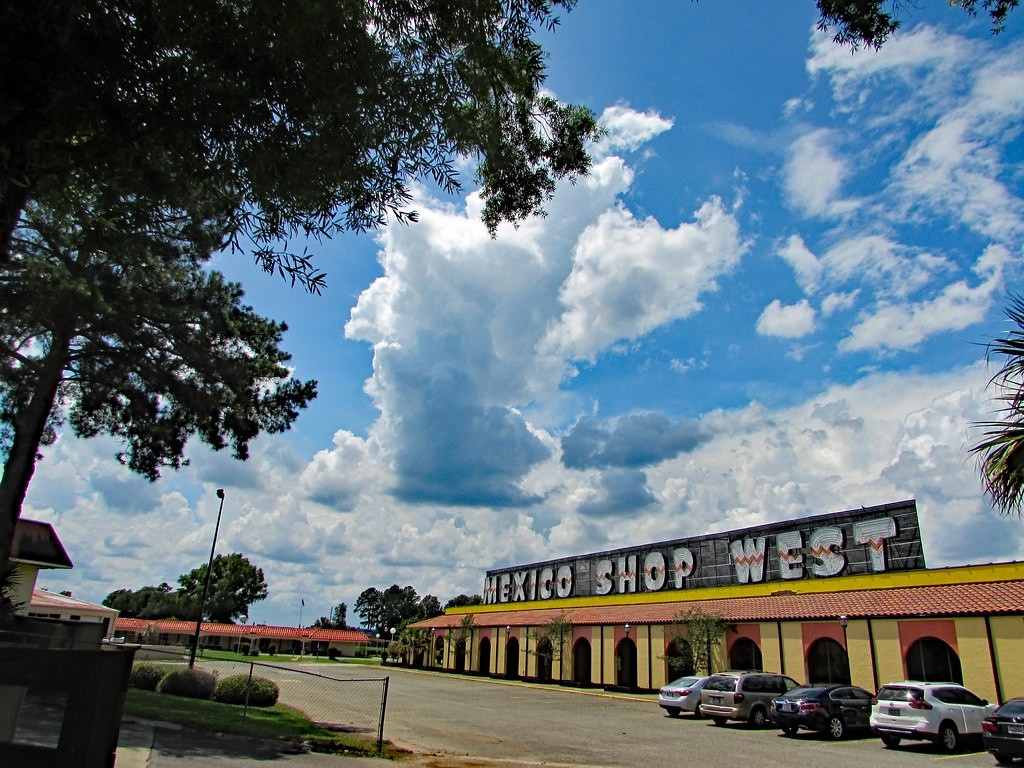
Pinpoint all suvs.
[869,680,1000,754]
[699,669,801,730]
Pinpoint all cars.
[658,676,712,720]
[981,697,1024,766]
[769,683,876,741]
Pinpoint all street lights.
[624,621,632,664]
[189,488,226,670]
[506,625,511,657]
[237,615,246,653]
[839,613,851,687]
[376,633,380,657]
[384,626,388,653]
[390,627,397,642]
[430,627,436,669]
